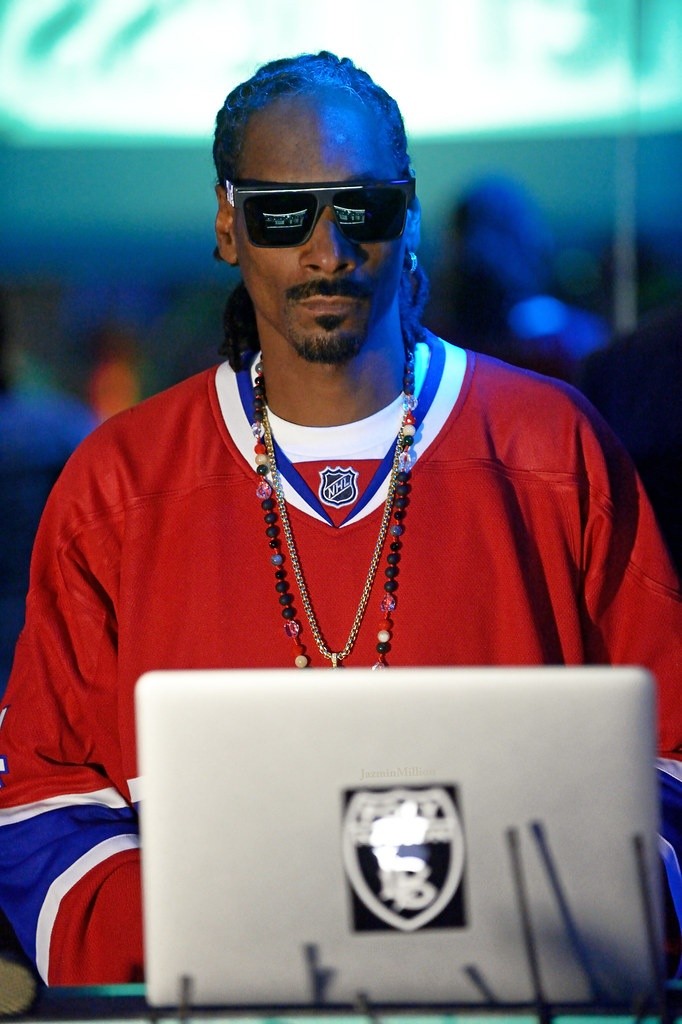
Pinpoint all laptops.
[134,666,667,1017]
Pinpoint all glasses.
[217,172,418,246]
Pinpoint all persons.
[1,47,682,984]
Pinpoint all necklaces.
[262,405,405,671]
[251,340,415,671]
